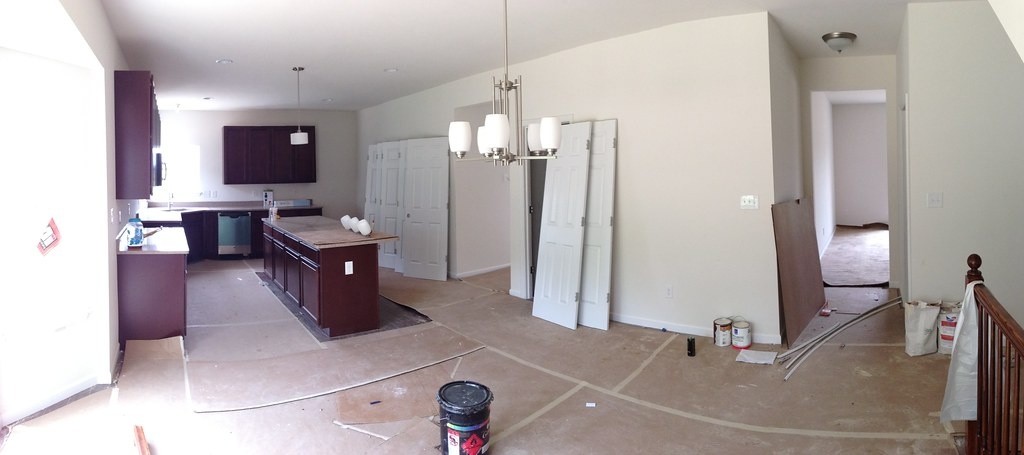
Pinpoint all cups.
[269,207,278,221]
[687,336,695,357]
[341,215,371,236]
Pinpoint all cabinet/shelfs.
[138,199,323,265]
[114,70,167,199]
[117,226,190,352]
[262,215,380,339]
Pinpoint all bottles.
[127,218,143,248]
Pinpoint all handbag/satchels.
[905,301,940,357]
[938,302,961,355]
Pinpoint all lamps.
[341,215,352,229]
[290,67,308,144]
[358,218,371,235]
[349,216,359,233]
[822,32,857,53]
[448,0,562,166]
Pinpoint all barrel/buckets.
[713,316,751,349]
[430,380,495,455]
